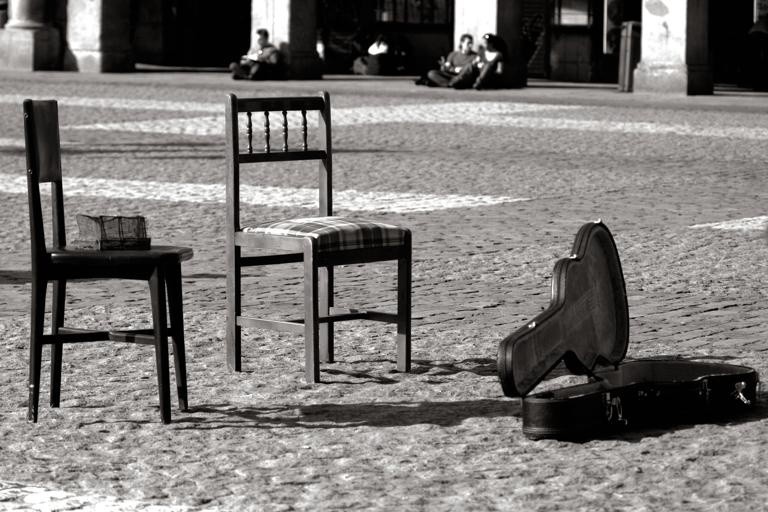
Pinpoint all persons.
[447,33,510,90]
[426,34,477,87]
[228,28,279,80]
[366,33,389,69]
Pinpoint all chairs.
[24,91,193,425]
[224,87,412,387]
